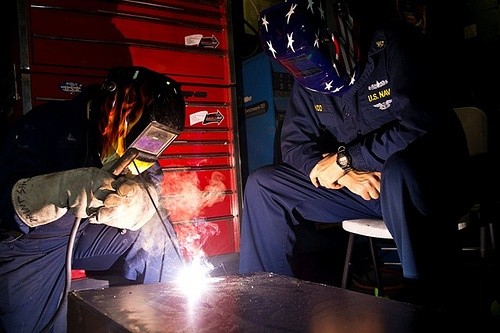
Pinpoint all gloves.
[85,176,158,232]
[11,168,118,227]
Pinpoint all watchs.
[336,142,354,173]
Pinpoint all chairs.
[340,107,488,289]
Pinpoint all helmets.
[95,65,186,179]
[259,0,367,95]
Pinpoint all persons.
[0,66,185,333]
[238,0,472,333]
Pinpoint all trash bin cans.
[354,269,409,301]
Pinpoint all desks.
[65,272,500,333]
